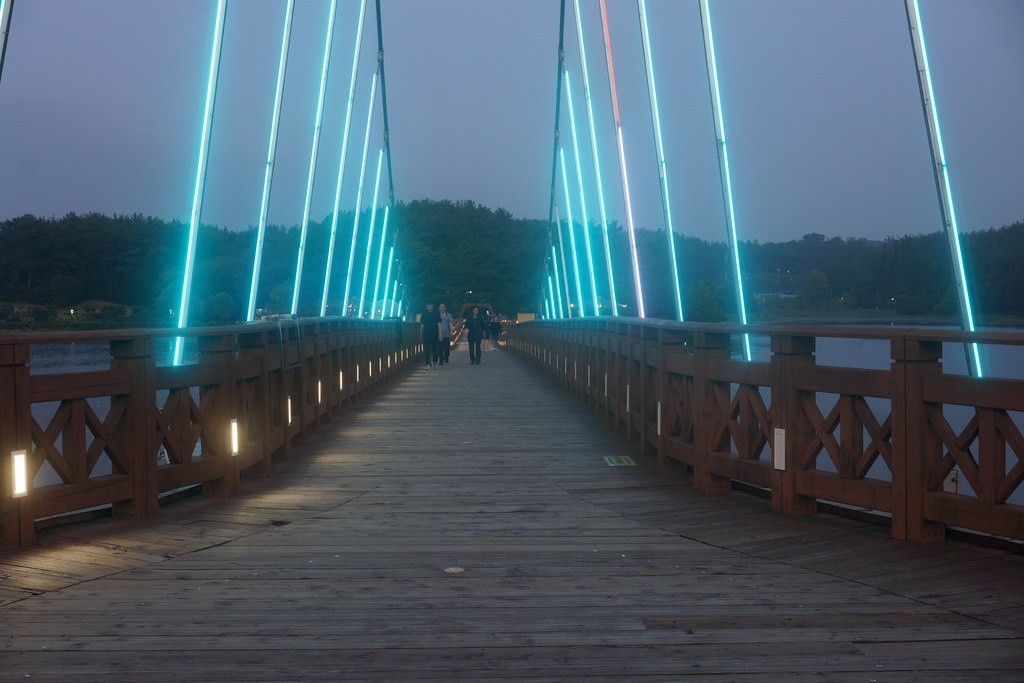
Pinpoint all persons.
[438,303,453,365]
[420,300,443,371]
[465,306,484,365]
[481,310,492,352]
[490,315,502,351]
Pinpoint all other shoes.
[493,348,497,350]
[471,360,476,364]
[445,358,449,363]
[433,362,438,370]
[426,364,432,370]
[439,360,445,365]
[476,359,480,364]
